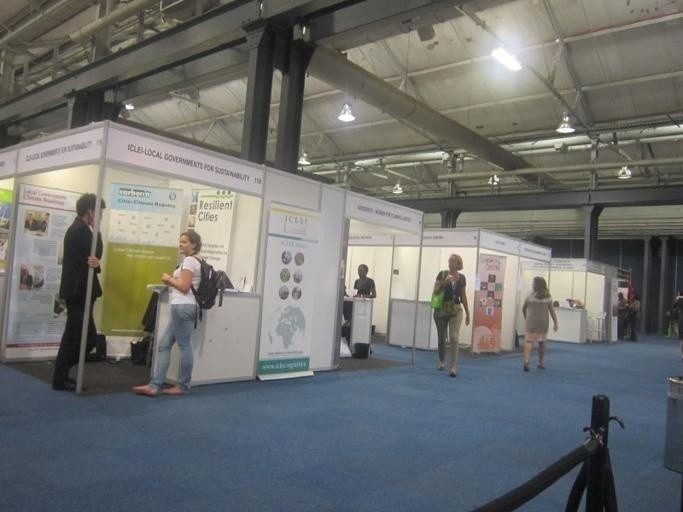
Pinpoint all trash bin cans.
[664,376,683,473]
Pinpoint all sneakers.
[524,360,546,372]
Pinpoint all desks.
[389,301,433,348]
[149,286,259,382]
[347,296,375,355]
[548,305,586,343]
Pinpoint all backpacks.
[180,254,234,309]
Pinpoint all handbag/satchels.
[430,270,446,309]
[130,330,147,364]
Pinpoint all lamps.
[299,152,311,167]
[337,101,356,123]
[393,183,403,194]
[616,165,632,180]
[490,45,527,71]
[488,176,500,188]
[555,116,576,135]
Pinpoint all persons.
[626,294,641,342]
[40,214,50,232]
[481,275,502,316]
[20,269,33,289]
[352,263,376,355]
[430,253,471,377]
[52,194,107,392]
[342,287,350,336]
[522,277,559,372]
[553,301,560,307]
[666,290,683,362]
[292,286,301,299]
[25,213,40,230]
[566,299,573,307]
[617,292,627,340]
[132,229,204,397]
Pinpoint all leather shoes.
[133,384,157,397]
[163,386,187,395]
[439,362,457,377]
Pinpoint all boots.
[53,367,88,391]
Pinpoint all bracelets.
[166,276,171,282]
[465,310,469,316]
